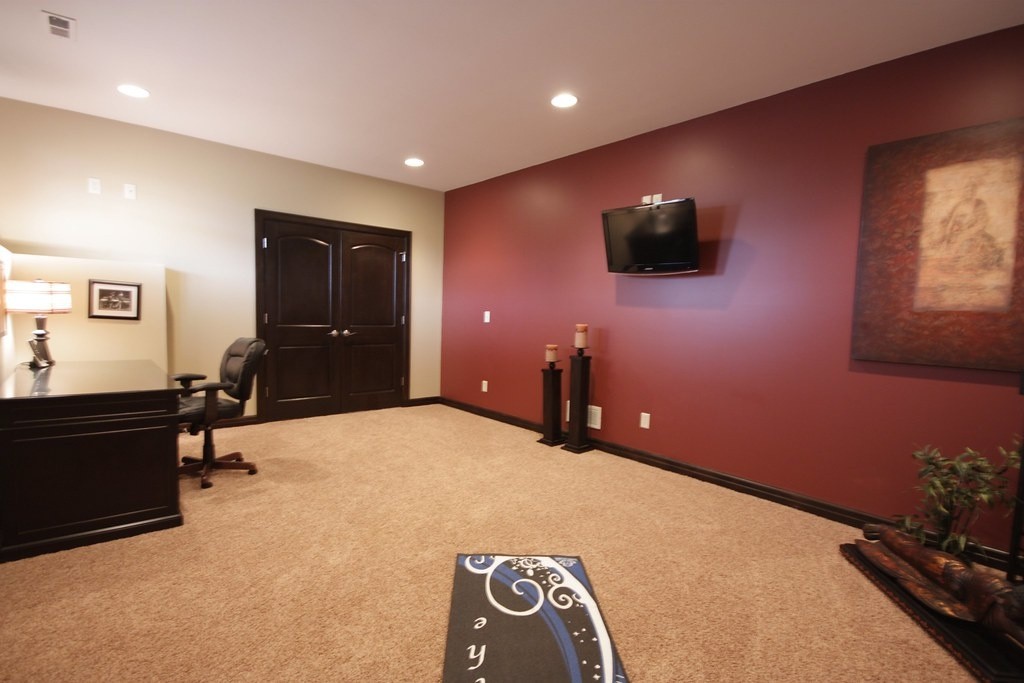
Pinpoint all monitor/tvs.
[601,197,700,276]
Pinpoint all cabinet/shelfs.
[0,358,185,563]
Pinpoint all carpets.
[441,553,632,683]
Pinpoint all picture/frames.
[852,113,1023,375]
[88,279,141,320]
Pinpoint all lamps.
[5,278,72,368]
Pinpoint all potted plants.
[854,440,1024,645]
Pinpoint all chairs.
[175,338,270,488]
